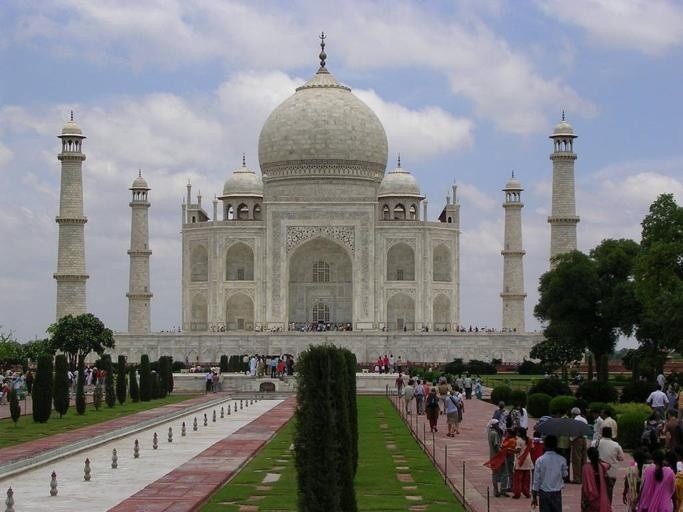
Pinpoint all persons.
[395,373,480,438]
[288,320,352,332]
[380,320,517,332]
[378,354,403,374]
[209,324,227,332]
[0,347,136,408]
[219,354,294,379]
[482,366,682,512]
[491,401,520,438]
[160,326,183,333]
[205,369,224,394]
[254,325,282,332]
[404,359,441,377]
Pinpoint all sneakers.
[406,411,447,415]
[494,488,531,499]
[430,426,459,437]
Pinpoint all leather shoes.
[569,481,578,484]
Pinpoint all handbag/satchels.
[604,472,613,492]
